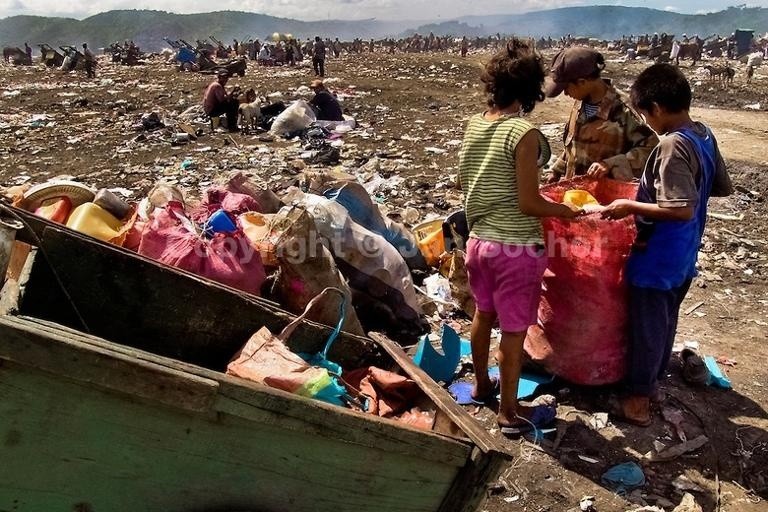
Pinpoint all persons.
[83,42,95,78]
[233,36,342,77]
[540,46,660,180]
[203,68,241,133]
[306,79,344,121]
[597,62,734,428]
[453,42,586,435]
[24,42,32,65]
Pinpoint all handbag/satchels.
[225,287,347,405]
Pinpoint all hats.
[310,80,322,88]
[218,69,229,78]
[542,47,606,97]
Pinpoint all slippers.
[601,396,651,427]
[471,376,500,406]
[501,405,557,434]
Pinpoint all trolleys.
[35,41,139,71]
[158,28,255,78]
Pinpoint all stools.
[193,112,217,131]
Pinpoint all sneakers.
[680,349,710,383]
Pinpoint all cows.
[671,43,697,66]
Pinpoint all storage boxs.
[0,200,514,512]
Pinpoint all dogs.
[703,63,735,83]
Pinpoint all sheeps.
[745,51,764,78]
[237,95,267,135]
